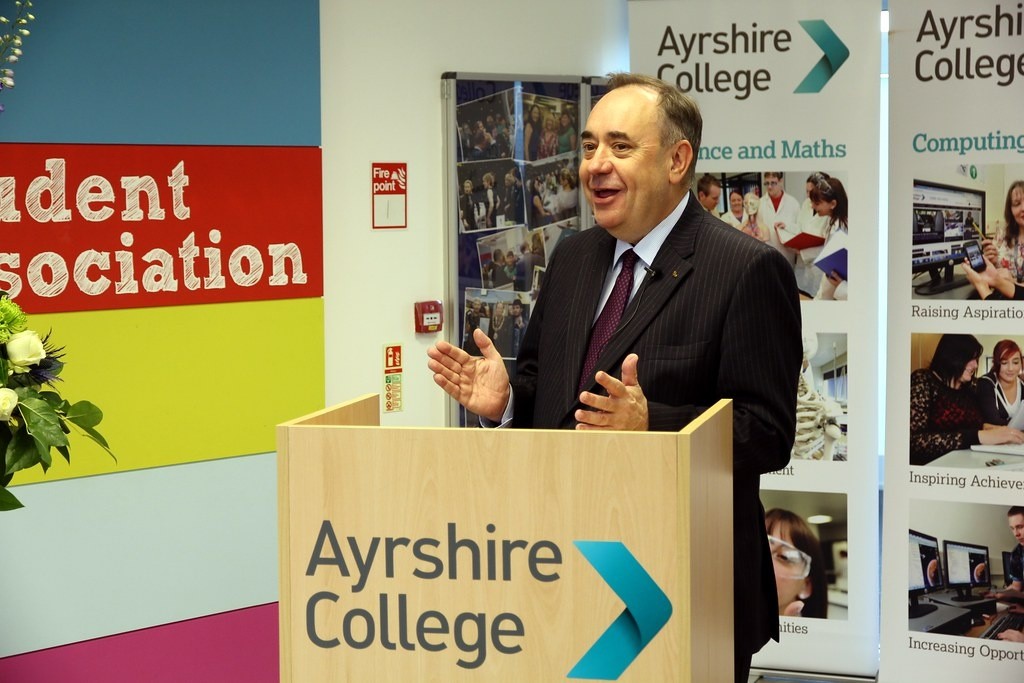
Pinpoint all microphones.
[645,265,662,281]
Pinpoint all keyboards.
[978,611,1024,642]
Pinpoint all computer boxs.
[908,593,998,634]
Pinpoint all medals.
[492,332,498,340]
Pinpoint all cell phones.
[962,241,986,273]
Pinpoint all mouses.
[1006,606,1015,612]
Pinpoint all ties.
[577,249,640,400]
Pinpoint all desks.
[912,264,975,300]
[923,450,1024,473]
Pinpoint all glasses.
[767,532,813,580]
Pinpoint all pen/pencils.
[972,223,987,240]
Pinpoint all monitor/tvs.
[943,540,992,602]
[908,528,945,618]
[1002,551,1013,587]
[912,179,987,295]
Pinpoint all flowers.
[0,0,117,512]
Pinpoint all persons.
[457,103,1024,645]
[427,73,804,683]
